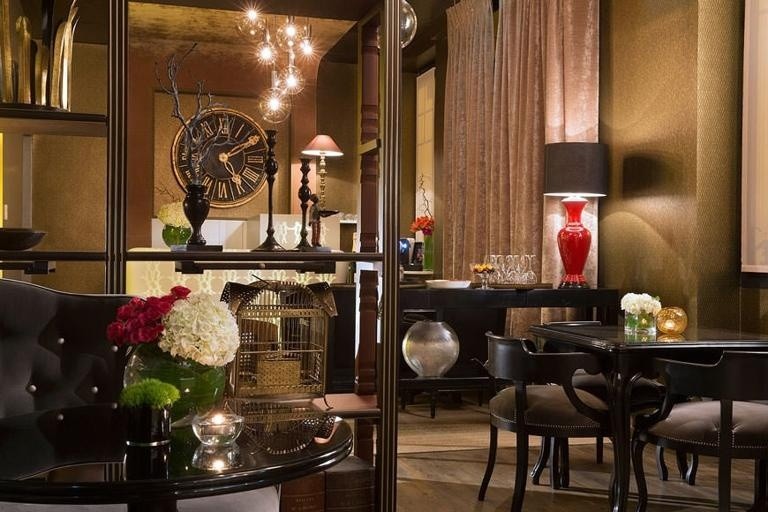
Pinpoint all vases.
[162,225,190,248]
[124,347,226,425]
[421,233,435,270]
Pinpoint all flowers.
[118,378,181,444]
[107,288,240,414]
[621,291,661,341]
[154,201,191,247]
[410,173,436,271]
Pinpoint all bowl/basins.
[191,443,245,470]
[0,225,46,253]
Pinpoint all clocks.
[170,107,270,210]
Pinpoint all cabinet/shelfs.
[399,288,618,324]
[1,0,398,509]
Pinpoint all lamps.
[543,143,605,288]
[236,15,321,124]
[658,308,688,336]
[302,133,344,211]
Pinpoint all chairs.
[632,352,768,512]
[477,332,613,512]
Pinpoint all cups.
[487,254,538,285]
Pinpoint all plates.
[424,278,472,289]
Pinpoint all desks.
[401,379,509,419]
[0,417,351,512]
[528,328,768,512]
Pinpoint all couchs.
[0,274,280,512]
